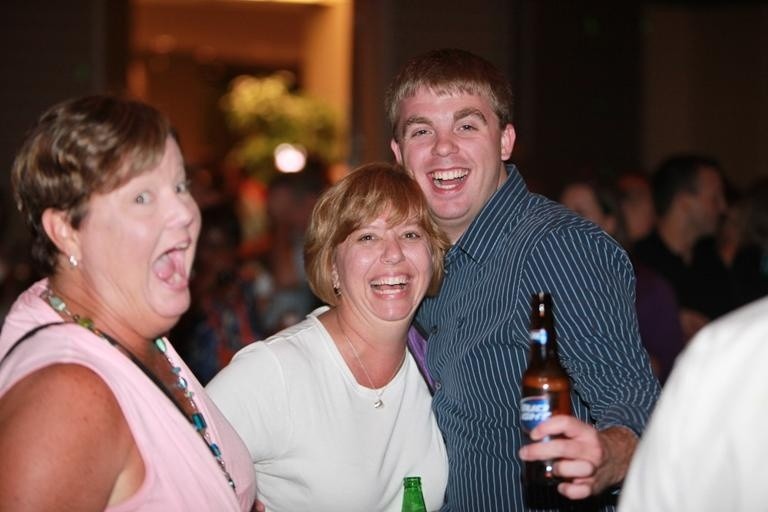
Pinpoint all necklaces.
[41,288,237,494]
[341,324,408,410]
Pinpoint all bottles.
[400,475,425,511]
[518,291,579,506]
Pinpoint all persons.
[562,150,767,381]
[198,161,453,510]
[614,290,766,512]
[389,52,665,511]
[163,157,332,382]
[0,90,270,511]
